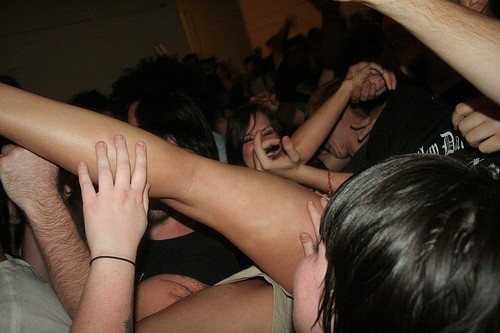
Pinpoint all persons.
[0,0,500,333]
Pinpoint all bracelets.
[327,170,334,195]
[89,255,136,269]
